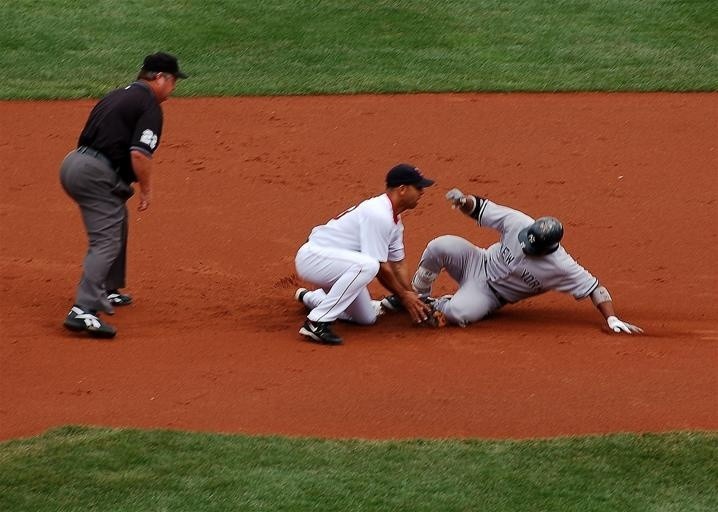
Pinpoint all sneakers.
[294,287,309,302]
[64,304,116,337]
[381,289,454,328]
[106,290,133,306]
[298,318,343,345]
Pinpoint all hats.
[386,164,434,189]
[141,51,189,79]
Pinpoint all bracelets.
[467,194,486,220]
[591,285,613,307]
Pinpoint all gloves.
[445,188,467,210]
[607,315,644,335]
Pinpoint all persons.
[382,186,645,336]
[55,52,189,338]
[293,159,431,347]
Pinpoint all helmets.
[518,217,564,257]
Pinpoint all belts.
[76,145,124,178]
[484,258,507,305]
